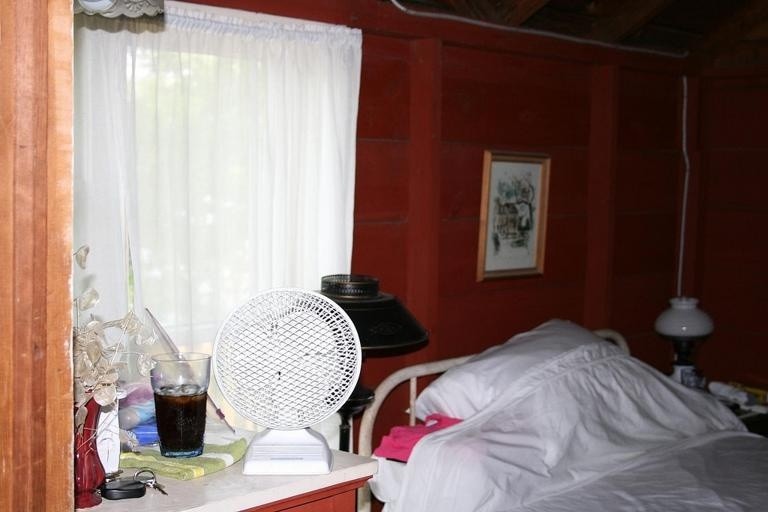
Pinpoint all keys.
[98,465,168,500]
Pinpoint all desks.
[74,450,379,511]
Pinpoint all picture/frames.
[476,148,551,283]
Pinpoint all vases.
[74,392,105,509]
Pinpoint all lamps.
[315,274,430,453]
[654,74,717,342]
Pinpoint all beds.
[358,328,768,511]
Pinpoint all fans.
[213,288,363,478]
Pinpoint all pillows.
[405,316,605,419]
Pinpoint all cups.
[149,352,212,458]
